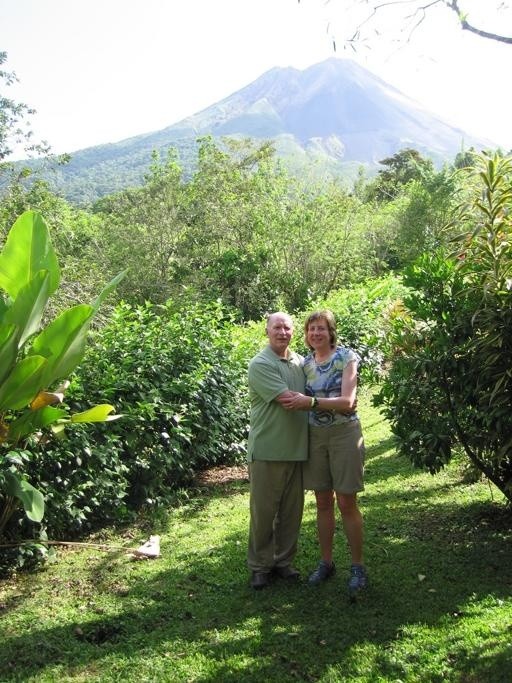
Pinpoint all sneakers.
[251,569,268,590]
[348,563,368,592]
[308,560,336,586]
[272,564,300,579]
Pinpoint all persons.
[248,310,369,588]
